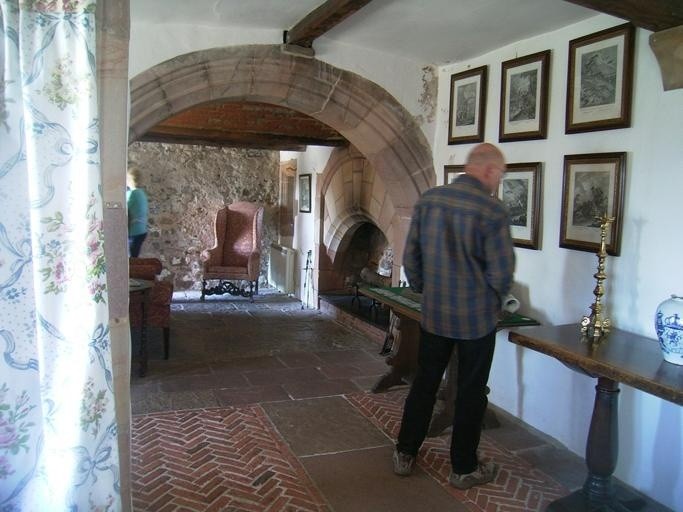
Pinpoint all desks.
[128,278,154,378]
[358,286,540,438]
[507,322,683,511]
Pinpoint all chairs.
[199,200,264,304]
[128,258,173,359]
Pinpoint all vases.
[653,295,683,367]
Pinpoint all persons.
[127,168,149,258]
[392,142,517,489]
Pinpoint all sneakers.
[450,463,497,491]
[394,448,416,477]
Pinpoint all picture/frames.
[443,151,627,257]
[447,22,635,146]
[298,173,311,213]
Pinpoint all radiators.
[267,242,295,294]
